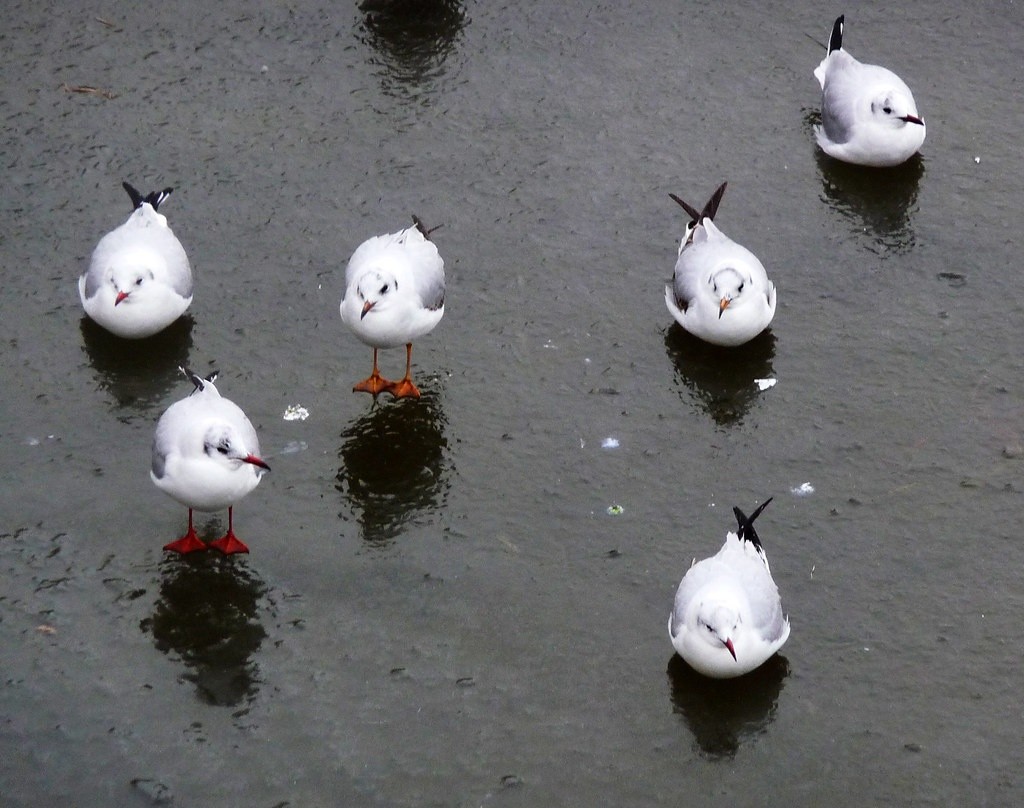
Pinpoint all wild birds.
[338,212,446,400]
[76,183,193,340]
[662,180,777,348]
[812,12,926,165]
[668,496,792,681]
[149,364,271,559]
